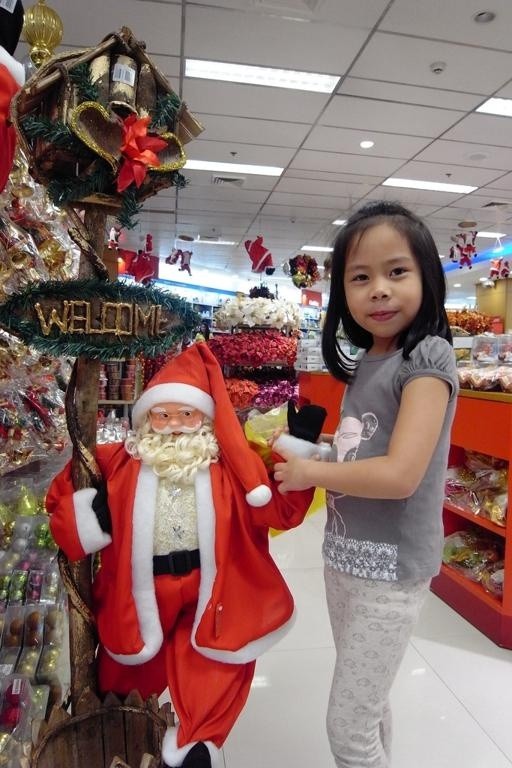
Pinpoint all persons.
[273,201,462,767]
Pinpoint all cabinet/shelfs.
[430,381,512,653]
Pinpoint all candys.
[0,485,64,768]
